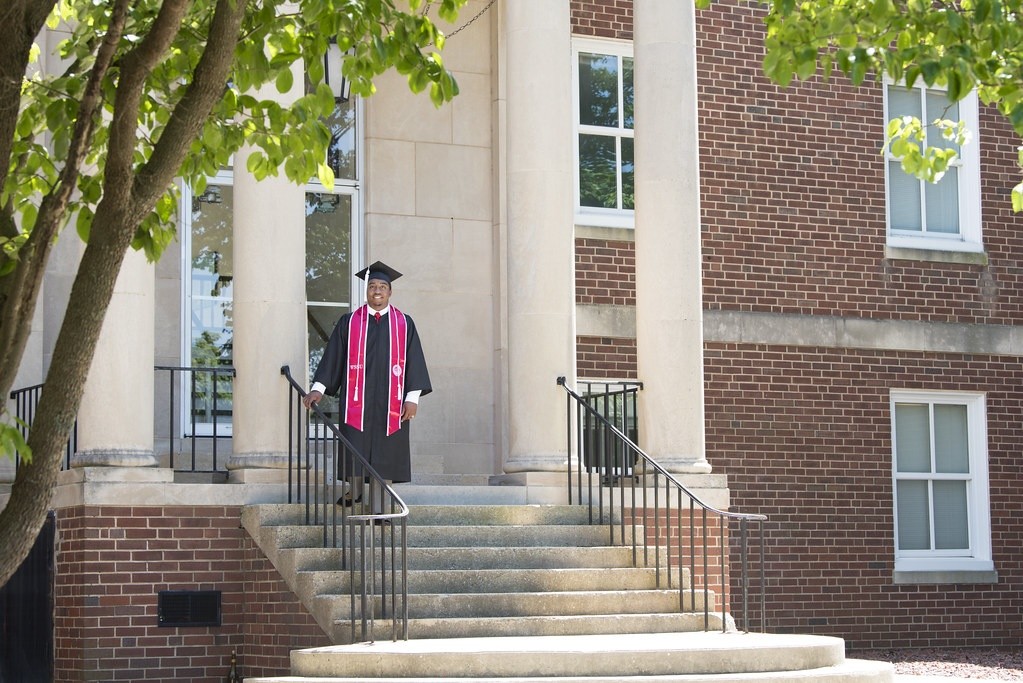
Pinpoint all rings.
[408,415,414,419]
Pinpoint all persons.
[303,260,433,525]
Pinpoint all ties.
[374,312,382,322]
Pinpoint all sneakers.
[337,491,363,507]
[375,513,391,526]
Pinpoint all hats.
[355,260,403,302]
